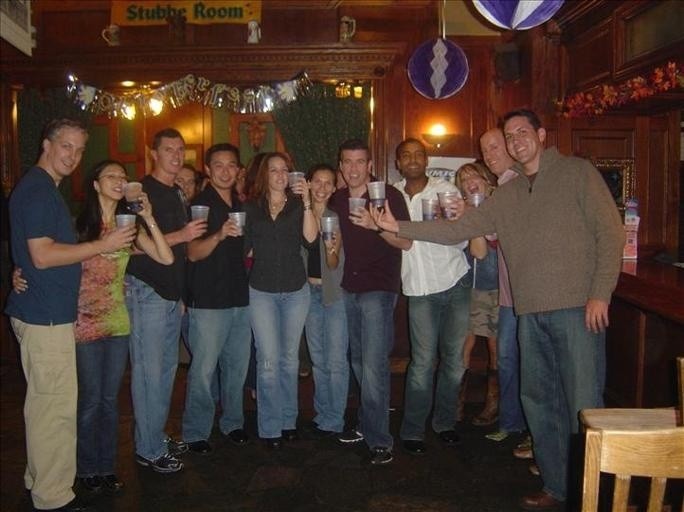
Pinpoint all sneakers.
[98,475,125,497]
[367,446,393,464]
[31,494,113,512]
[160,439,189,456]
[79,478,106,495]
[512,446,534,459]
[516,439,532,448]
[135,452,185,474]
[481,431,512,443]
[335,431,366,448]
[529,463,540,476]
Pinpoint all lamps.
[424,124,455,147]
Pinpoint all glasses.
[98,174,129,181]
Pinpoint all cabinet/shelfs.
[606,291,683,410]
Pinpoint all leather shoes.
[519,491,566,512]
[221,429,249,446]
[439,430,460,448]
[303,430,335,442]
[402,440,425,456]
[267,438,281,449]
[282,430,297,439]
[185,442,208,456]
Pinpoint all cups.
[116,214,136,231]
[320,216,338,242]
[287,172,304,198]
[123,182,144,213]
[366,181,386,208]
[191,205,209,224]
[467,194,484,207]
[436,191,459,218]
[247,19,261,43]
[421,198,439,222]
[103,24,123,46]
[228,212,246,236]
[338,16,356,42]
[348,198,366,221]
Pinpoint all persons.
[3,109,624,511]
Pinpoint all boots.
[471,369,499,426]
[455,367,471,424]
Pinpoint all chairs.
[577,408,684,511]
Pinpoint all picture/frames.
[593,156,636,212]
